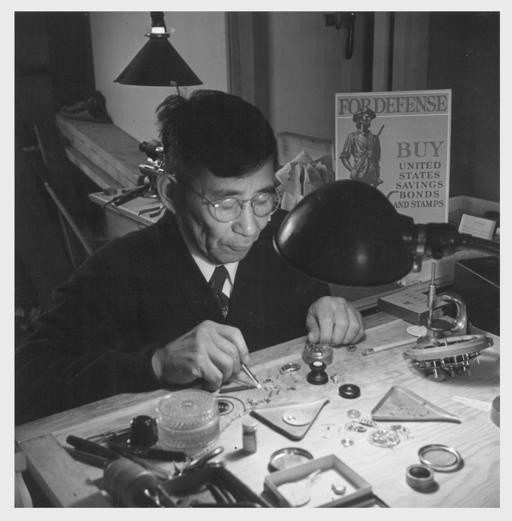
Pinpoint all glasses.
[173,174,280,222]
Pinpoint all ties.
[208,265,230,322]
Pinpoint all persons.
[339,107,381,188]
[16,90,365,394]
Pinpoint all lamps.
[273,180,500,427]
[114,11,205,84]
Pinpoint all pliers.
[64,435,170,481]
[138,204,165,218]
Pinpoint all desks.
[13,311,499,508]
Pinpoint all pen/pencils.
[362,337,419,356]
